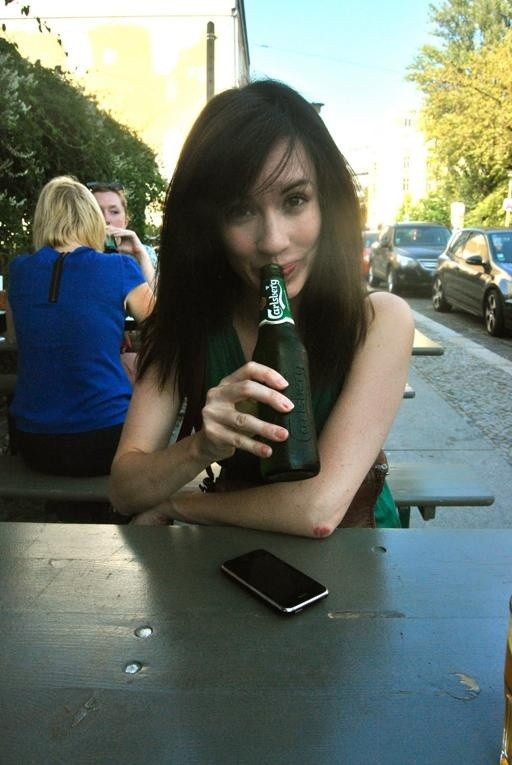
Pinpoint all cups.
[498,596,511,764]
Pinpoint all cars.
[361,222,512,336]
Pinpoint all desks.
[0,327,444,356]
[0,521,512,765]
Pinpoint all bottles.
[104,234,118,253]
[251,263,321,483]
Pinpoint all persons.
[104,78,418,543]
[0,174,158,479]
[87,181,160,304]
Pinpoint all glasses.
[87,182,126,201]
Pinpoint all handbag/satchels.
[207,449,389,529]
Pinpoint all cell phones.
[220,548,329,617]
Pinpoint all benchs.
[0,454,495,528]
[403,383,415,398]
